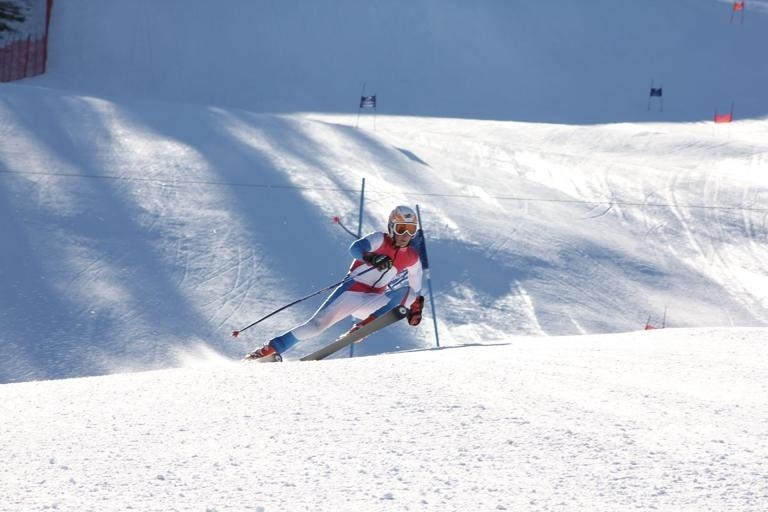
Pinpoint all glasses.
[388,220,417,237]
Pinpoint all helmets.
[387,206,417,240]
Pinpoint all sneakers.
[248,345,277,359]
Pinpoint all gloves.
[407,302,423,326]
[371,254,393,271]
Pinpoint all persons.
[239,202,427,360]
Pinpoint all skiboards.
[248,305,410,362]
